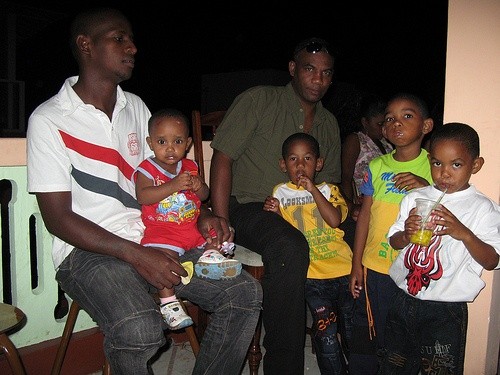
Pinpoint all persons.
[341,102,394,221]
[379,123,500,375]
[131,110,242,330]
[263,132,355,375]
[210,37,340,375]
[348,94,435,375]
[27,7,264,375]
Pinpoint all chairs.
[0,303,28,375]
[50,301,201,375]
[191,111,318,375]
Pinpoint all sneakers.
[195,252,242,281]
[160,300,193,330]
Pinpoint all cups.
[410,198,442,247]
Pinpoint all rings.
[228,227,234,232]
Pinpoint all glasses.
[292,40,340,57]
